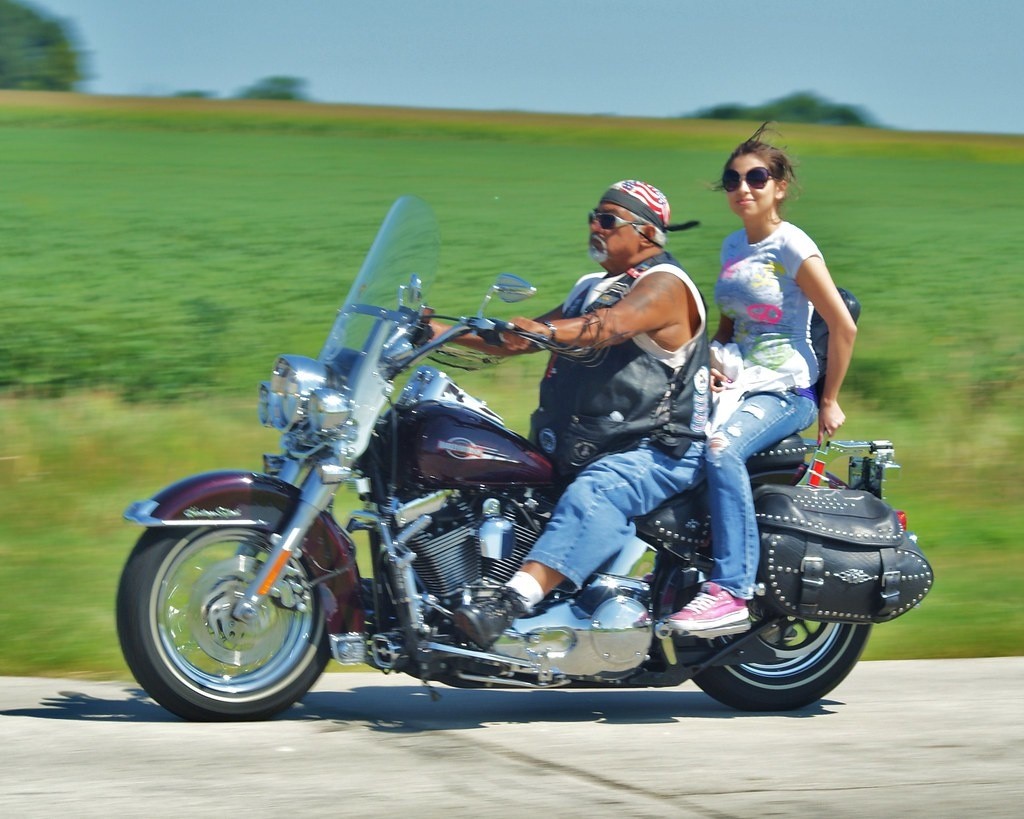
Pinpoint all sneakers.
[454,587,531,649]
[666,582,749,628]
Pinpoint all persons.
[428,180,710,650]
[600,121,858,630]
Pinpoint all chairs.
[811,285,860,408]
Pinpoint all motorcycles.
[117,194,936,721]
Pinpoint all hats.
[605,179,699,233]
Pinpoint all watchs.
[543,320,557,336]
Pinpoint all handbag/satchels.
[753,482,934,625]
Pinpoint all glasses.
[722,167,781,192]
[588,211,647,229]
